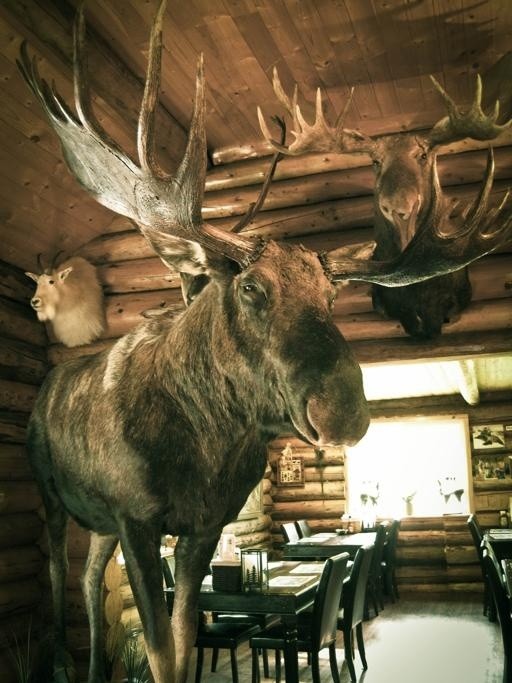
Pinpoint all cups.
[219,534,235,562]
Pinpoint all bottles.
[406,502,413,516]
[347,515,354,534]
[499,514,508,529]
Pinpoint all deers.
[256,65,512,341]
[27,1,512,683]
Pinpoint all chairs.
[466,510,512,683]
[160,518,401,683]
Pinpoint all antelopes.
[25,248,106,348]
